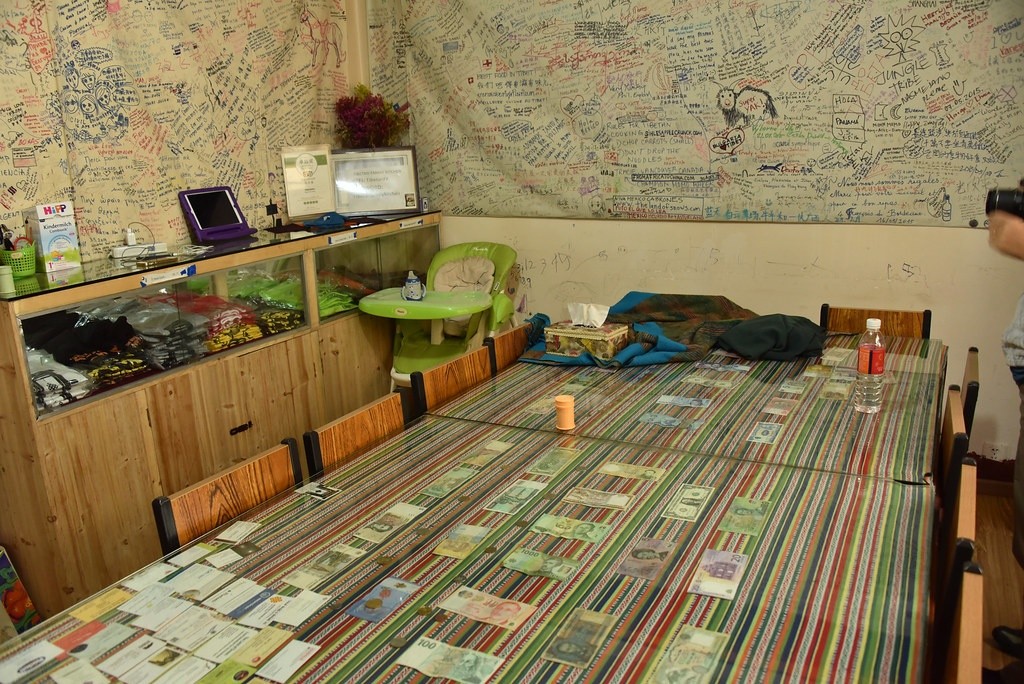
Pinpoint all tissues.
[543,302,629,362]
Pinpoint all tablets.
[179,186,257,242]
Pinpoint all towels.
[515,290,760,368]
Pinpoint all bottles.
[853,318,886,414]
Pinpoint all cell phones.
[137,257,177,267]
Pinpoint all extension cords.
[111,243,167,258]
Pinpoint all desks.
[0,333,950,684]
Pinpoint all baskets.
[0,245,36,277]
[14,277,40,296]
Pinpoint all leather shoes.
[982,666,1003,684]
[992,626,1024,659]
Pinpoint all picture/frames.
[331,145,421,217]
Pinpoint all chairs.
[921,347,983,684]
[359,241,518,393]
[410,322,530,418]
[152,437,303,556]
[302,389,412,481]
[819,304,931,339]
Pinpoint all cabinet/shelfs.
[0,208,442,620]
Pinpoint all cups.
[401,277,427,302]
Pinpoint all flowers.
[333,82,410,153]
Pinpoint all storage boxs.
[0,546,40,637]
[22,201,81,273]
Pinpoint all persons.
[989,210,1024,389]
